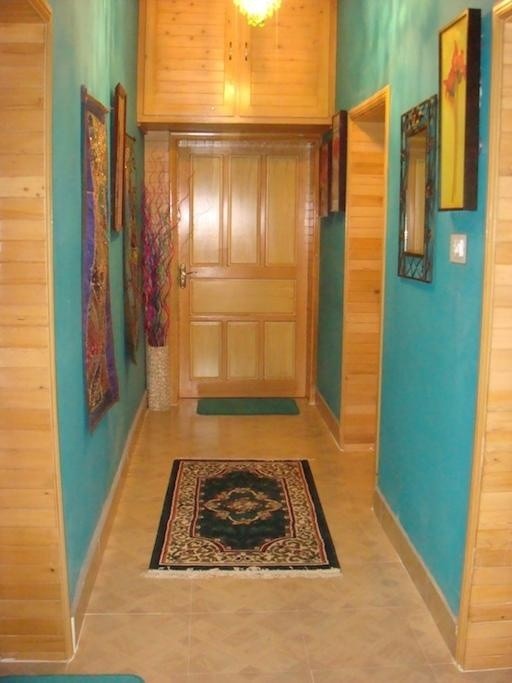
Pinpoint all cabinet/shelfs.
[137,0,338,133]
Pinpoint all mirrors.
[396,94,437,284]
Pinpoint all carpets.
[198,396,300,415]
[147,459,341,578]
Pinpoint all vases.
[146,345,172,414]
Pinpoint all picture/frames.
[327,111,347,217]
[115,82,127,231]
[433,6,481,211]
